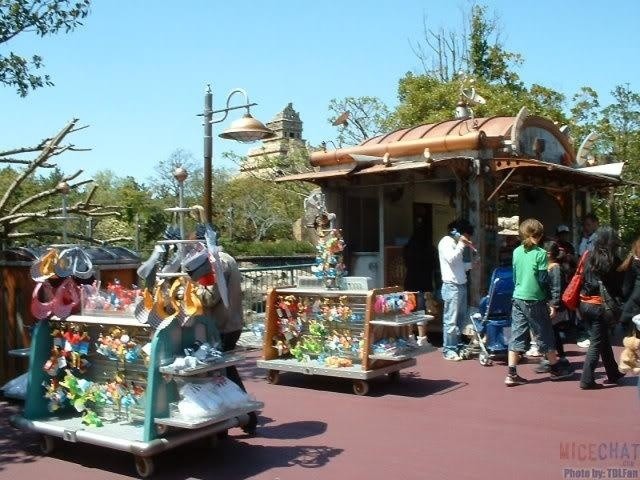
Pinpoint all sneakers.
[505,375,529,386]
[443,349,464,363]
[409,335,417,348]
[535,363,552,374]
[239,415,258,431]
[416,336,433,350]
[548,367,575,381]
[459,348,470,359]
[578,338,590,349]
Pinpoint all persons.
[576,214,601,348]
[504,218,576,387]
[576,226,628,390]
[616,239,640,339]
[533,240,573,374]
[555,223,576,312]
[437,219,481,362]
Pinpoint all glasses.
[557,248,565,252]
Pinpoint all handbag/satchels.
[562,250,589,312]
[594,272,623,326]
[424,291,441,321]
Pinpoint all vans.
[45,244,148,265]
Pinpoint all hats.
[554,224,572,235]
[196,222,221,239]
[52,280,78,318]
[414,215,427,229]
[184,281,203,317]
[31,281,54,319]
[30,250,57,281]
[56,247,75,279]
[186,244,209,270]
[154,279,181,319]
[162,252,181,278]
[138,244,166,280]
[159,225,186,240]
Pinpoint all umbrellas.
[190,205,233,312]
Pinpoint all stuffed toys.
[618,335,640,377]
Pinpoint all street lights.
[196,82,279,225]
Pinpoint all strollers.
[460,267,513,365]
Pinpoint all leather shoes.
[578,382,604,390]
[609,374,626,384]
